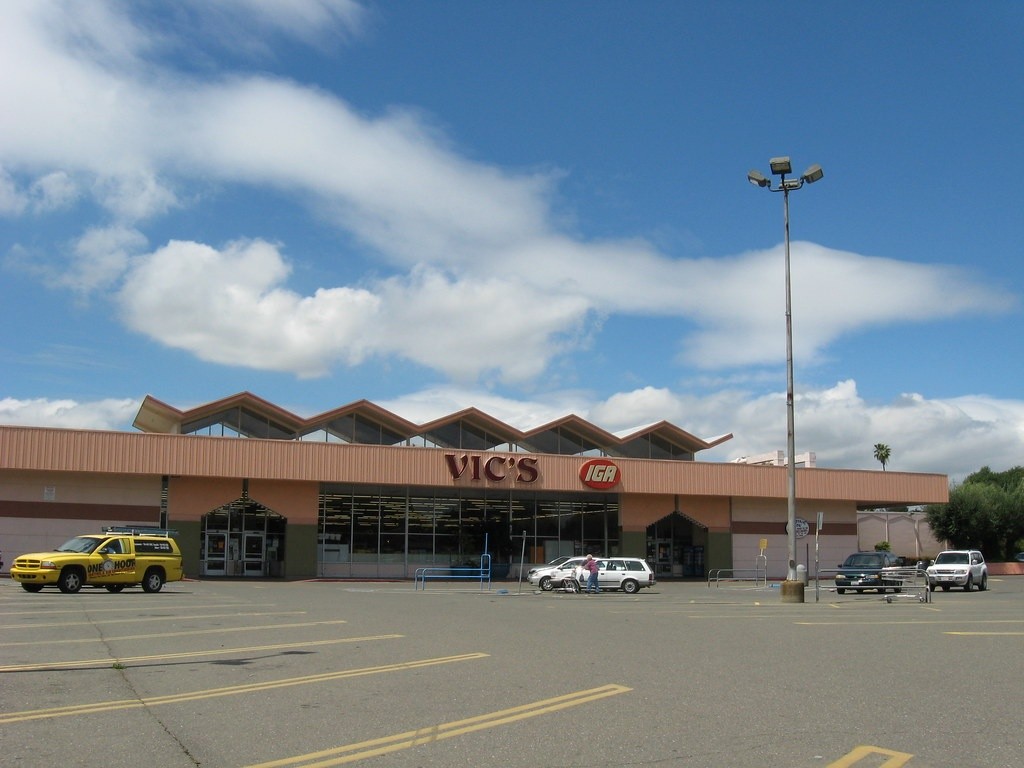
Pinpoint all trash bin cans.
[795,563,808,587]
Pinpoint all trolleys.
[552,566,580,594]
[882,561,926,604]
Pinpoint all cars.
[527,556,657,593]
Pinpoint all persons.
[581,555,600,594]
[104,544,116,554]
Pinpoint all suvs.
[835,550,903,594]
[926,549,988,592]
[9,526,185,595]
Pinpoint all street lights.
[745,155,824,603]
